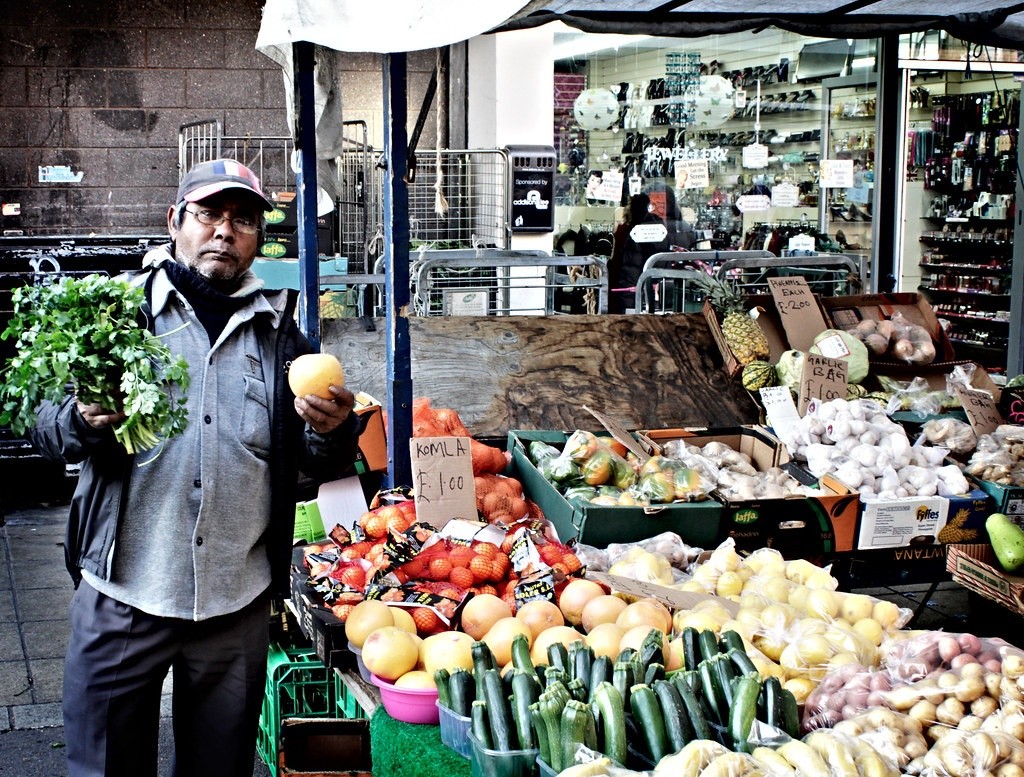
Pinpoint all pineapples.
[690,260,772,363]
[938,508,977,543]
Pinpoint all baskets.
[254,643,333,777]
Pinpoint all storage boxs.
[254,290,1023,777]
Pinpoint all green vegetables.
[0,273,191,468]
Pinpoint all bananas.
[552,727,901,777]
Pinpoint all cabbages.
[808,328,870,384]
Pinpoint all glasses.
[178,205,262,235]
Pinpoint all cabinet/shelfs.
[914,88,1019,374]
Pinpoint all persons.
[18,158,360,777]
[611,191,672,316]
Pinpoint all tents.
[251,0,1024,517]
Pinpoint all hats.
[176,158,274,216]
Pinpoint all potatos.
[833,656,1024,777]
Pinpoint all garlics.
[790,395,970,498]
